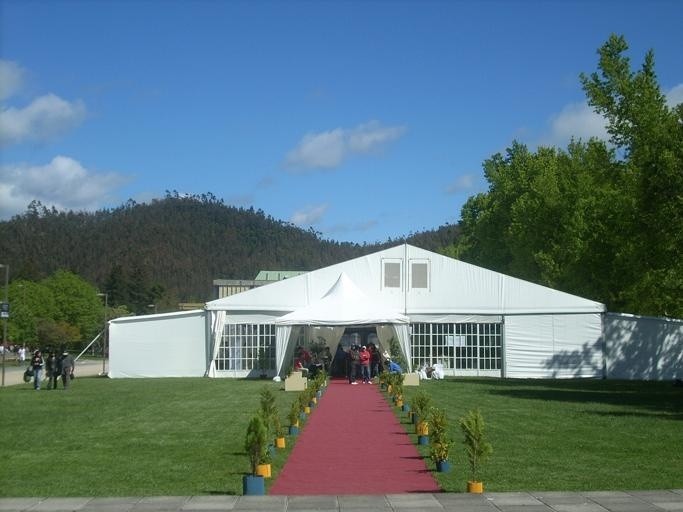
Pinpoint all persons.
[0,341,39,367]
[31,349,74,390]
[294,343,402,385]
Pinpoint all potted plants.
[307,390,318,407]
[304,398,312,413]
[393,385,403,407]
[255,429,273,479]
[387,374,393,393]
[402,396,409,412]
[244,414,266,495]
[312,371,328,399]
[457,407,494,493]
[274,407,287,449]
[289,410,300,435]
[430,408,451,472]
[379,372,388,389]
[298,403,307,420]
[410,395,431,446]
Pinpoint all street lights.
[0,264,10,385]
[96,293,108,376]
[148,304,157,313]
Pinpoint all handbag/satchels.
[23,369,34,383]
[69,373,74,380]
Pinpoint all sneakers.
[34,385,57,391]
[350,380,372,385]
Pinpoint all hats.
[362,346,366,349]
[351,343,356,346]
